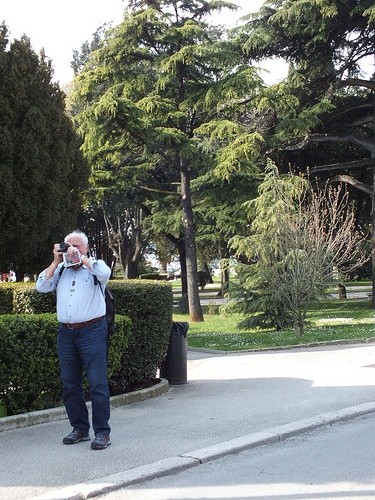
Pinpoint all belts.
[59,314,106,329]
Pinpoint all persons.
[35,232,112,450]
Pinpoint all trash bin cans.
[159,322,190,386]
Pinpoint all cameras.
[58,242,70,252]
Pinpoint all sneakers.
[63,427,91,445]
[90,434,111,450]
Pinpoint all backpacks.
[55,261,116,335]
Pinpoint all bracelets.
[80,254,85,262]
